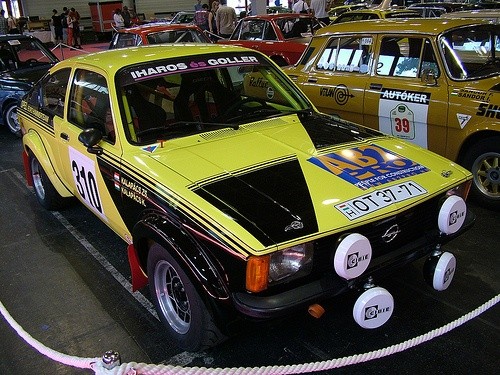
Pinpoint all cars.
[108,1,500,66]
[242,18,499,214]
[18,42,479,352]
[0,33,62,136]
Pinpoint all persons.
[121,6,130,29]
[113,8,125,30]
[0,9,9,35]
[6,11,18,34]
[193,0,376,43]
[52,6,82,50]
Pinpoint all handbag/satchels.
[300,3,307,13]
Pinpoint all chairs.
[133,105,167,127]
[214,91,242,109]
